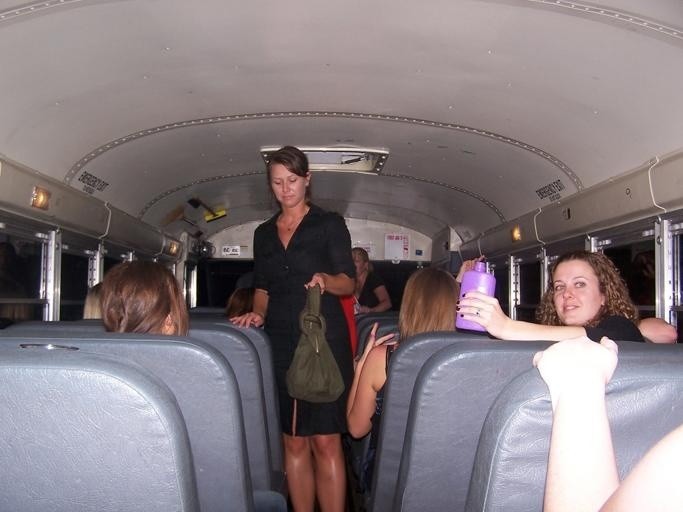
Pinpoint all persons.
[83,259,190,336]
[532,337,683,512]
[456,251,678,343]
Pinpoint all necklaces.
[280,207,309,231]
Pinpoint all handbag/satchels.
[285,283,345,403]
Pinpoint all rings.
[476,309,480,316]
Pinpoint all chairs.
[1,305,287,512]
[347,310,660,512]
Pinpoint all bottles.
[453,259,498,333]
